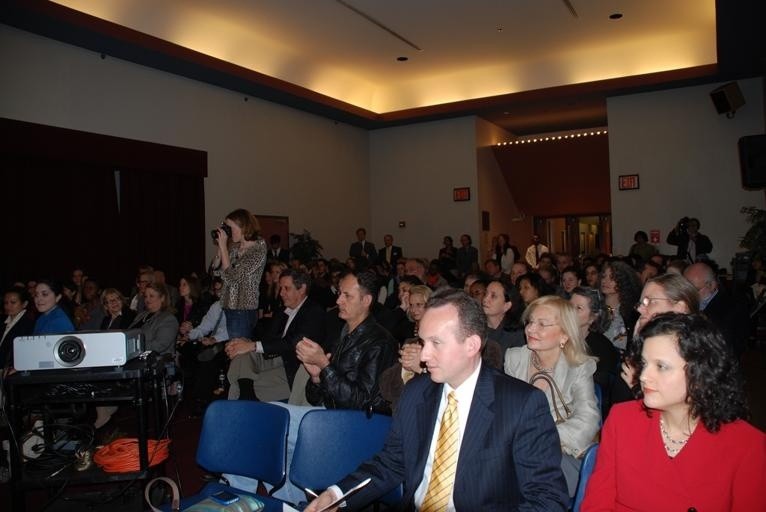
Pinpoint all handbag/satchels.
[529,371,585,499]
[175,339,200,368]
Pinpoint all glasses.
[634,295,670,309]
[525,317,560,328]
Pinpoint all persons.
[1,266,224,436]
[439,235,459,264]
[629,231,660,262]
[666,215,713,257]
[582,312,764,511]
[210,211,267,341]
[266,234,287,260]
[458,235,478,268]
[304,293,568,511]
[503,297,599,500]
[378,235,403,265]
[525,234,549,267]
[349,227,377,265]
[490,234,520,276]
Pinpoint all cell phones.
[208,490,240,506]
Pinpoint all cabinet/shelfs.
[6,358,184,512]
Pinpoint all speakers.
[710,82,747,116]
[738,134,766,191]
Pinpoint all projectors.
[12,328,147,372]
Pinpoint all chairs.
[289,409,403,512]
[154,400,290,512]
[595,384,602,427]
[573,443,600,512]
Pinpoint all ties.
[210,309,223,336]
[535,246,539,265]
[418,391,459,512]
[274,250,277,257]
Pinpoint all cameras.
[211,223,232,239]
[679,217,689,231]
